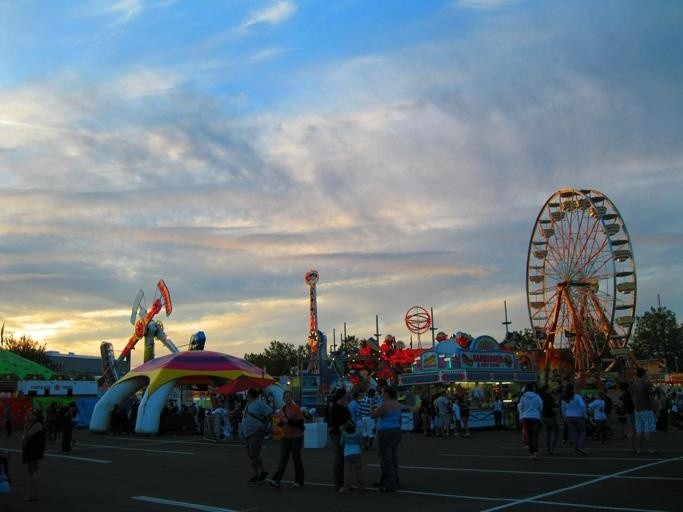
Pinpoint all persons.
[493,397,502,431]
[323,389,365,493]
[371,386,401,492]
[22,401,81,502]
[159,399,245,440]
[5,402,13,437]
[418,390,472,438]
[2,447,16,504]
[110,399,139,436]
[266,389,306,487]
[346,389,381,448]
[654,386,682,432]
[242,388,273,484]
[512,370,659,460]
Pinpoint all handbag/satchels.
[344,444,360,457]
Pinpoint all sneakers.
[248,471,279,487]
